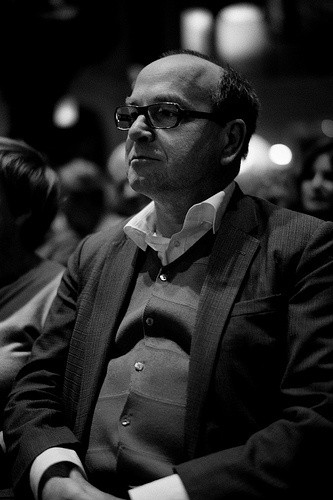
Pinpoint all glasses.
[114,102,232,131]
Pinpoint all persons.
[105,142,154,216]
[32,159,111,271]
[278,137,333,222]
[3,49,333,500]
[0,133,70,391]
